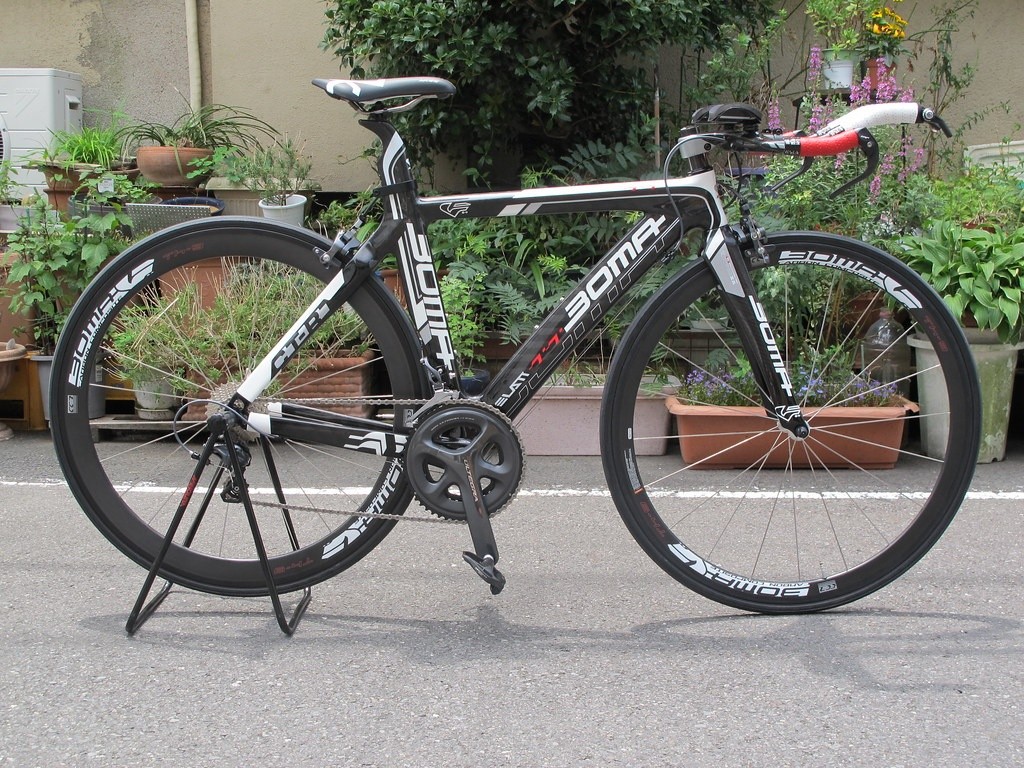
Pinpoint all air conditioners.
[0,67,83,204]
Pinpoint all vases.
[863,58,897,93]
[665,394,923,470]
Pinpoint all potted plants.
[479,0,1024,464]
[0,87,491,423]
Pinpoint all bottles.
[862,307,913,450]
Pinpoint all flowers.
[767,43,927,244]
[675,353,900,406]
[863,6,906,58]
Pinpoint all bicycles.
[46,71,986,643]
